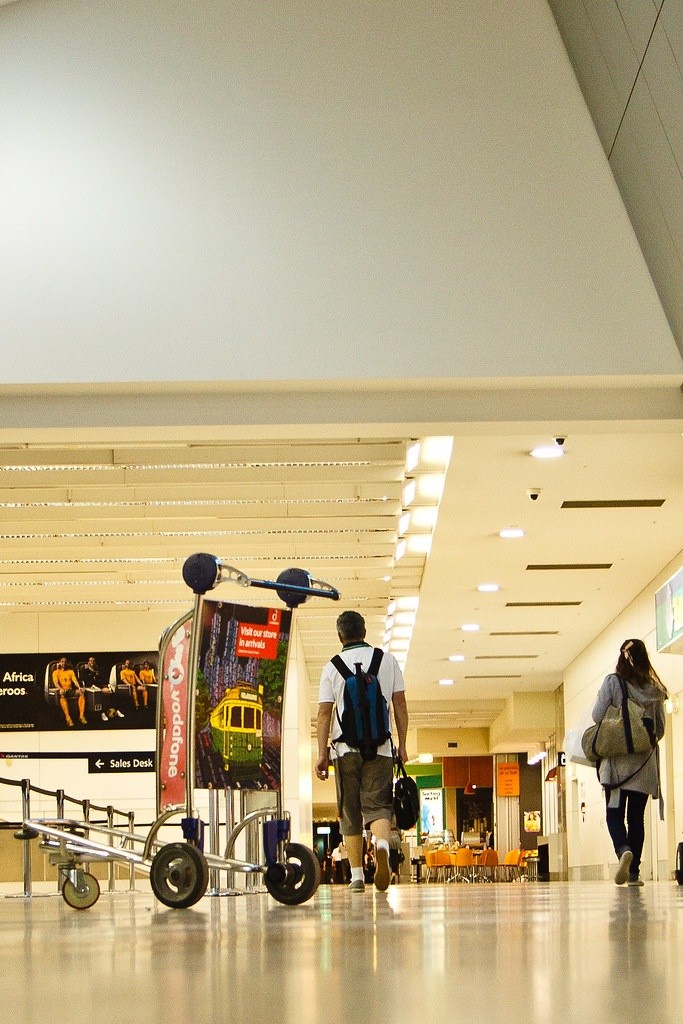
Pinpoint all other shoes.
[628,874,644,886]
[614,851,633,885]
[374,846,389,891]
[391,872,396,884]
[348,879,365,893]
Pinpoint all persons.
[120,660,158,711]
[591,639,667,886]
[315,611,409,891]
[52,657,124,727]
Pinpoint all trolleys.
[10,551,322,909]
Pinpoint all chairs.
[43,660,158,720]
[421,844,538,884]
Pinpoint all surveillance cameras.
[528,488,541,500]
[552,434,567,446]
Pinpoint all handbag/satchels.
[394,760,419,830]
[581,673,656,761]
[399,853,404,862]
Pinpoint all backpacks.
[366,852,374,870]
[331,648,391,748]
